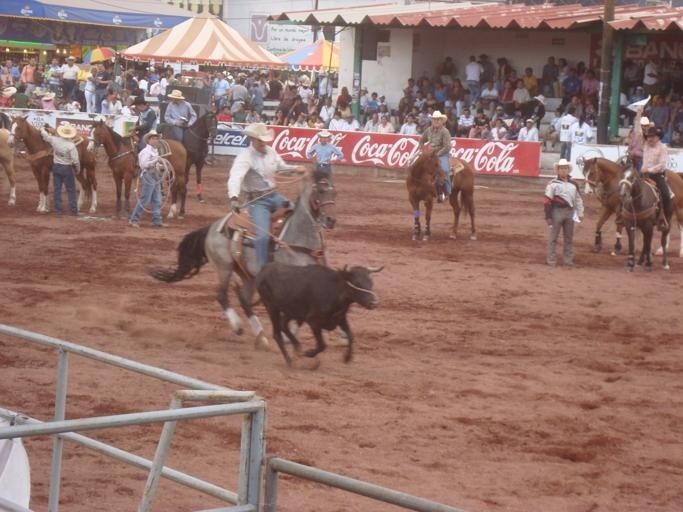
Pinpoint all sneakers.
[547,259,557,265]
[563,259,575,267]
[440,193,448,201]
[71,210,78,215]
[128,221,139,228]
[659,220,667,231]
[54,209,63,215]
[151,221,169,227]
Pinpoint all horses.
[86,117,188,220]
[156,108,220,203]
[583,157,683,259]
[0,128,17,207]
[618,157,673,273]
[0,112,11,130]
[147,162,350,352]
[6,115,98,214]
[406,148,477,241]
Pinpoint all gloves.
[231,196,240,215]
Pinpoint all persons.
[37,121,79,217]
[543,158,587,269]
[639,124,673,223]
[128,129,169,229]
[415,108,454,202]
[1,55,682,173]
[306,127,343,178]
[226,119,305,277]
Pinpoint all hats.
[641,117,655,127]
[56,124,76,138]
[146,67,155,71]
[554,159,573,173]
[345,114,354,118]
[223,71,234,79]
[144,130,162,143]
[167,90,185,100]
[318,130,331,138]
[428,111,447,123]
[242,123,275,142]
[527,119,536,123]
[318,73,326,76]
[361,87,369,93]
[42,92,55,100]
[535,95,544,105]
[64,56,78,61]
[130,96,147,105]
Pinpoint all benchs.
[451,81,632,168]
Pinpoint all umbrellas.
[81,45,117,65]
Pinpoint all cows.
[235,263,385,368]
